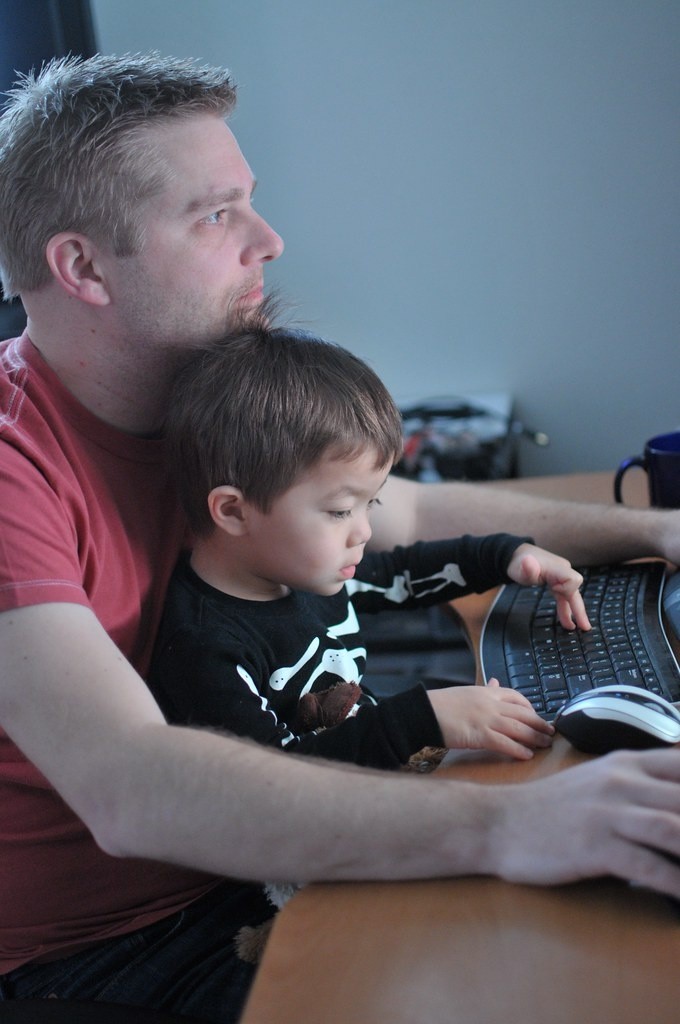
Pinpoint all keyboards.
[479,561,680,723]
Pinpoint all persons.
[0,56,680,1024]
[137,308,591,960]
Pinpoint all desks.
[235,467,679,1024]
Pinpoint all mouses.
[553,684,680,757]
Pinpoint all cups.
[614,431,680,509]
